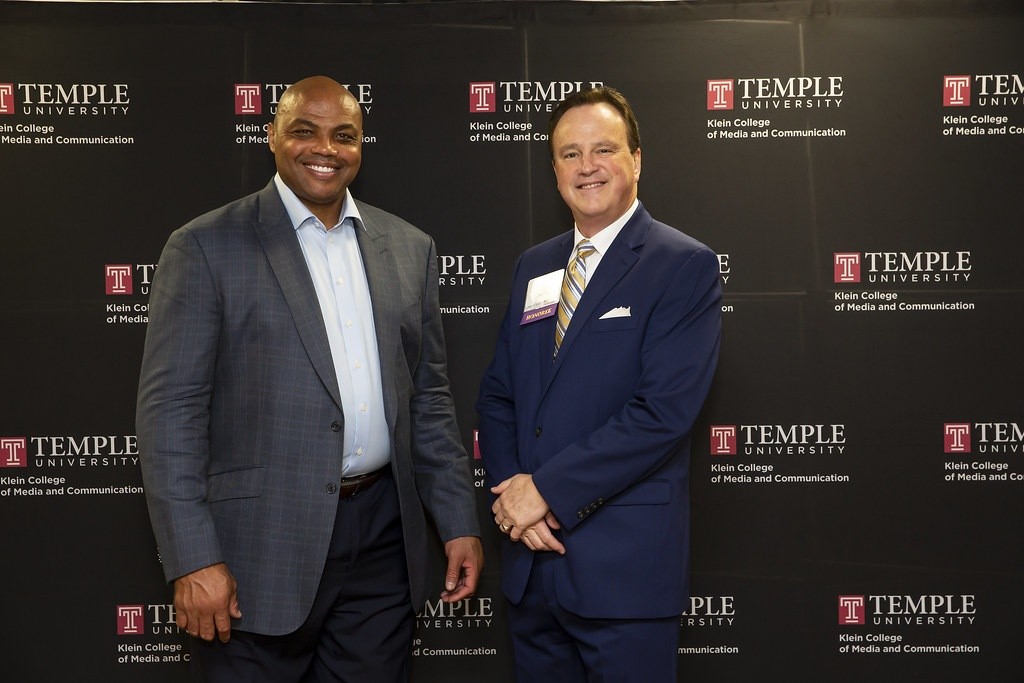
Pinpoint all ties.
[554,239,596,362]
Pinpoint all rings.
[501,523,510,531]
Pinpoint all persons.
[477,87,720,683]
[136,75,486,683]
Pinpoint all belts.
[339,466,392,500]
